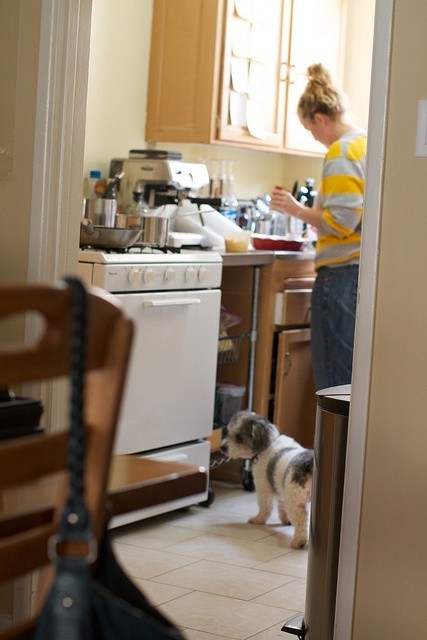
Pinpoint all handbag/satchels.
[34,533,189,637]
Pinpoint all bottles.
[103,185,118,227]
[86,171,101,225]
[125,190,152,216]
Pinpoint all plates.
[251,234,310,251]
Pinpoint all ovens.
[74,264,223,506]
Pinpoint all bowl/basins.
[225,232,252,252]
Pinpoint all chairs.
[0,280,138,640]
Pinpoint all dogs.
[219,409,315,550]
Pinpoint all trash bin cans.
[281,384,352,640]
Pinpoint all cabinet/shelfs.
[144,1,343,156]
[264,276,318,449]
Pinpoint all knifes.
[293,176,320,208]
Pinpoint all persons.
[269,63,368,392]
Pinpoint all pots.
[80,220,144,249]
[116,208,217,248]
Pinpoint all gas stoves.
[79,243,224,263]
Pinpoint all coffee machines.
[109,149,212,252]
